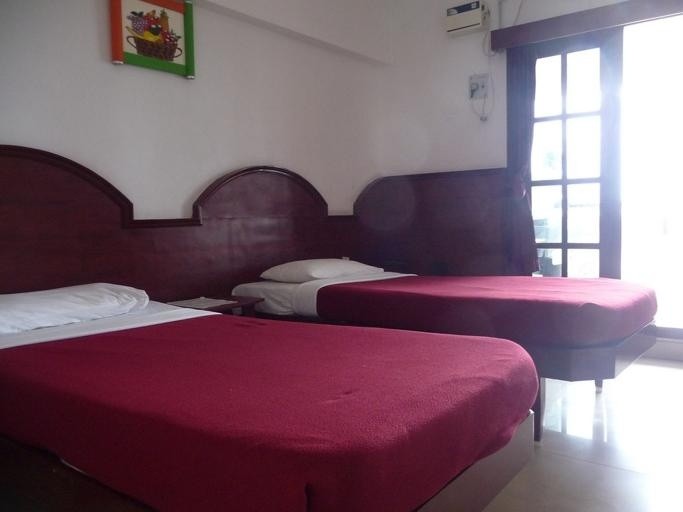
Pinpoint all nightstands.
[164,294,265,317]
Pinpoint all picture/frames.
[110,0,193,80]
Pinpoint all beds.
[230,271,656,440]
[1,301,544,512]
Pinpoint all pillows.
[259,256,385,282]
[1,282,150,335]
